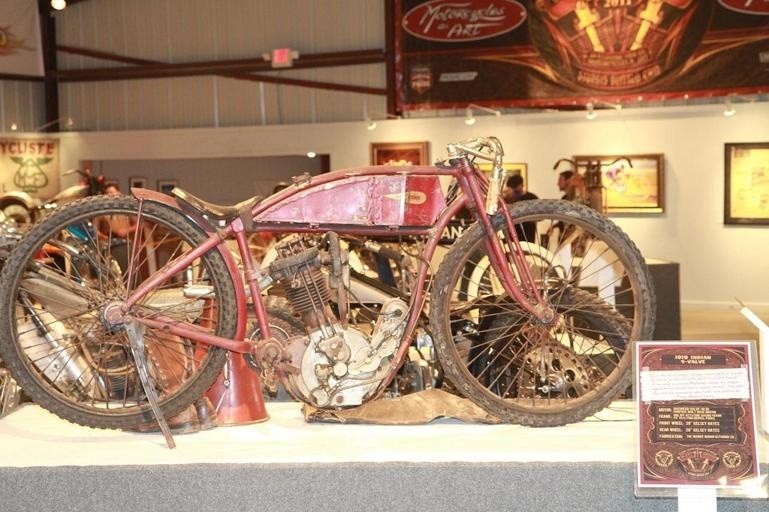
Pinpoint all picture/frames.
[369,140,430,167]
[571,153,666,216]
[128,176,177,197]
[471,163,529,196]
[722,141,769,226]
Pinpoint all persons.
[98,181,151,287]
[545,169,594,258]
[502,172,543,241]
